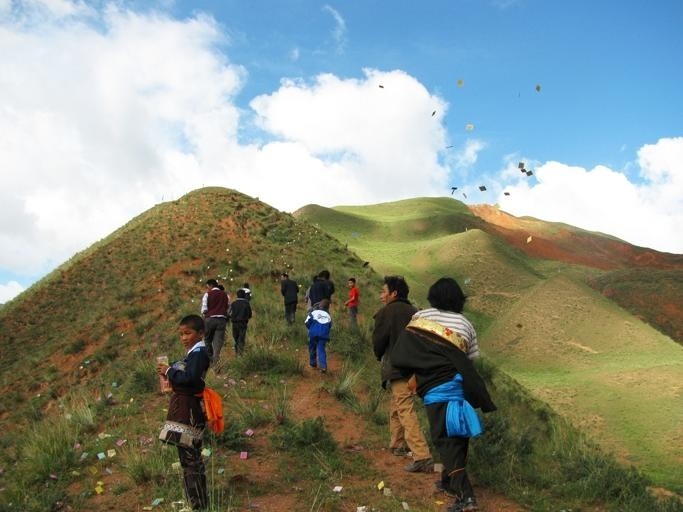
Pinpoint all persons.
[155,315,213,512]
[305,270,335,373]
[200,278,253,366]
[389,277,497,512]
[372,276,434,473]
[280,272,299,326]
[344,277,359,336]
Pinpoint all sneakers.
[434,480,456,497]
[448,498,477,511]
[404,457,434,472]
[390,448,412,457]
